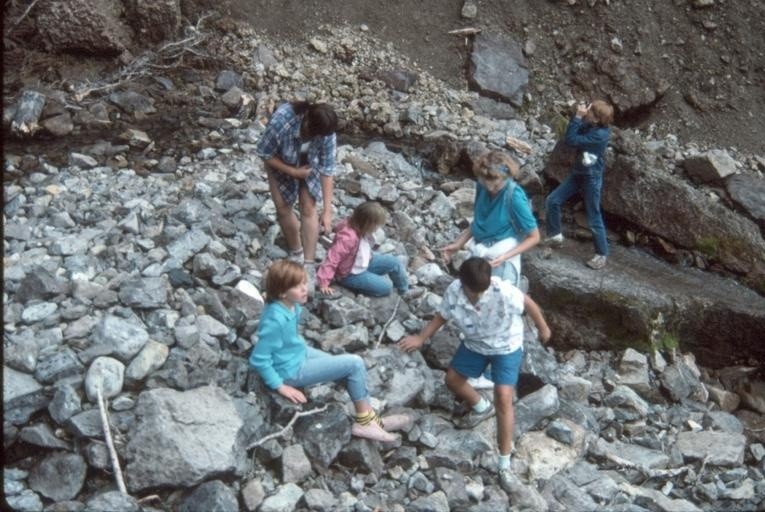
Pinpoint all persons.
[312,199,428,303]
[433,145,542,295]
[542,99,615,271]
[254,97,339,285]
[249,258,398,443]
[396,255,554,496]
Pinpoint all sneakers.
[302,265,316,293]
[399,285,428,304]
[467,374,494,389]
[455,398,495,429]
[585,253,606,270]
[351,419,395,442]
[536,238,564,249]
[496,465,520,494]
[287,247,304,266]
